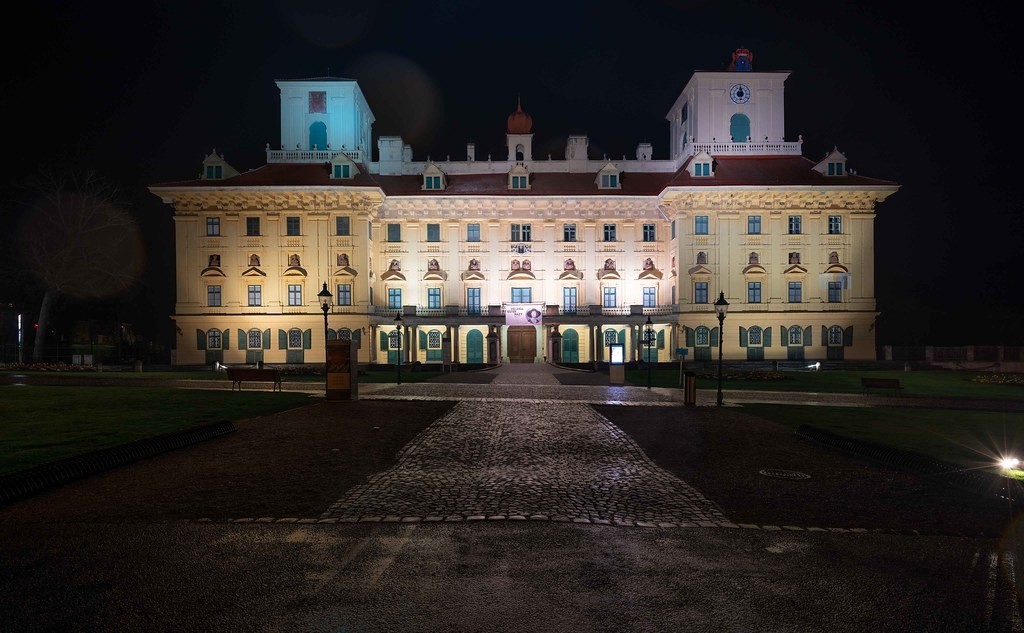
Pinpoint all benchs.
[227,366,282,392]
[860,377,904,394]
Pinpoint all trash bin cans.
[683,372,696,407]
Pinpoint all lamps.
[544,356,547,361]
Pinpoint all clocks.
[728,83,753,104]
